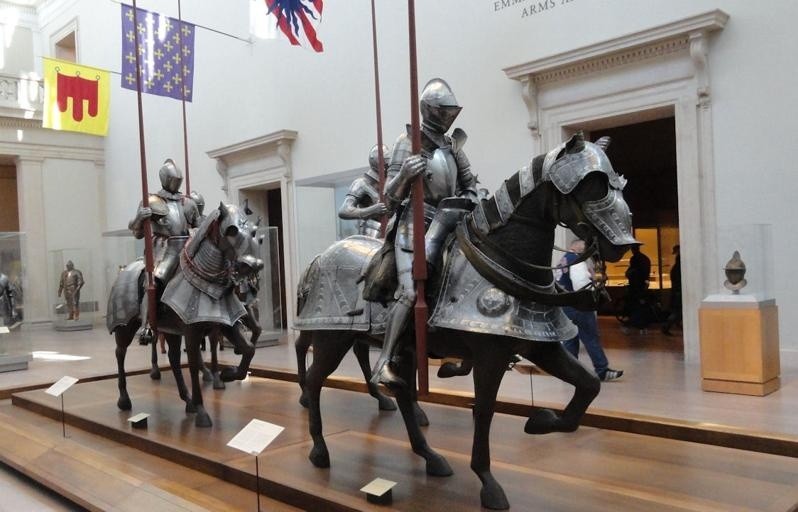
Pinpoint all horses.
[285,130,648,510]
[98,200,269,430]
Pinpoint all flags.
[249,0,325,54]
[40,56,111,138]
[120,2,195,103]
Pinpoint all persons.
[127,158,203,344]
[551,229,623,383]
[368,79,477,385]
[626,245,652,335]
[57,260,84,320]
[188,191,206,224]
[338,143,395,239]
[1,273,19,331]
[659,245,682,336]
